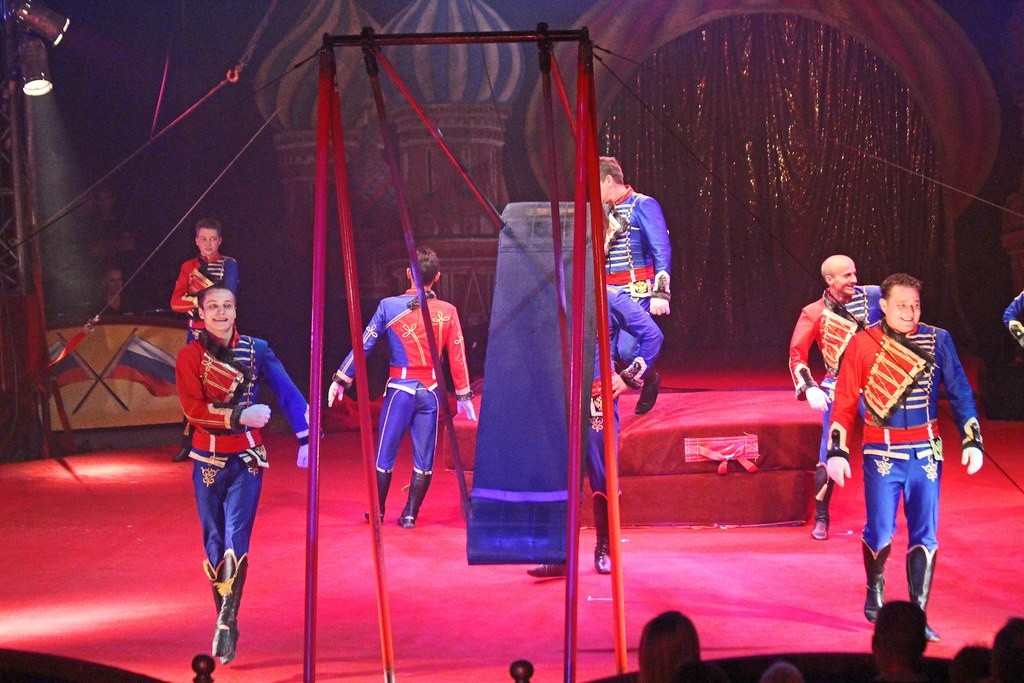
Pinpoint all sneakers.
[635,369,661,414]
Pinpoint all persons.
[329,247,476,527]
[789,255,883,540]
[174,282,323,664]
[524,158,673,576]
[635,600,1024,683]
[824,274,983,643]
[1002,289,1024,348]
[79,181,240,462]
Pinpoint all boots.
[172,435,196,462]
[591,491,622,575]
[203,559,240,664]
[365,467,393,524]
[811,461,835,540]
[527,564,566,576]
[397,466,433,528]
[905,544,941,642]
[211,549,249,657]
[861,537,892,623]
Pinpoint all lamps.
[11,2,69,45]
[15,30,54,97]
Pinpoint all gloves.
[826,457,851,488]
[297,444,308,468]
[239,404,271,428]
[806,387,831,414]
[650,297,671,316]
[961,447,983,474]
[328,381,344,407]
[457,399,476,420]
